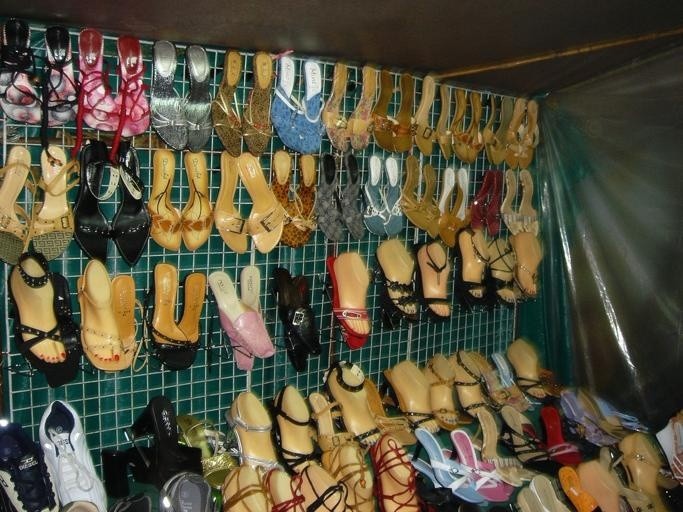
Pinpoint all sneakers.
[108,493,152,512]
[159,472,211,512]
[60,501,100,512]
[0,423,59,512]
[39,399,108,512]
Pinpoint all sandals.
[71,29,120,158]
[455,227,488,306]
[224,392,279,471]
[307,393,359,452]
[7,250,66,375]
[142,264,191,367]
[111,275,148,372]
[39,26,79,148]
[263,467,306,512]
[322,442,374,512]
[415,241,451,321]
[30,146,74,261]
[428,360,460,430]
[454,351,486,420]
[77,259,128,372]
[221,464,272,512]
[488,239,515,307]
[0,17,42,124]
[323,361,380,449]
[45,273,84,388]
[165,273,213,373]
[301,465,354,512]
[72,138,111,266]
[371,433,421,512]
[111,140,152,267]
[176,414,239,489]
[657,473,683,512]
[109,34,151,164]
[271,384,324,476]
[656,410,683,485]
[0,146,31,266]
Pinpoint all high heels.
[449,430,509,502]
[441,447,514,496]
[500,406,554,474]
[470,435,537,481]
[498,431,511,450]
[472,410,523,487]
[411,428,485,503]
[539,407,581,467]
[408,454,445,488]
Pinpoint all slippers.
[451,168,472,232]
[323,257,369,338]
[214,150,249,254]
[611,433,668,512]
[458,502,480,512]
[496,97,514,143]
[181,151,214,251]
[150,40,189,151]
[436,167,457,248]
[382,156,403,236]
[488,506,508,512]
[414,75,437,156]
[577,388,627,441]
[230,266,262,370]
[400,156,428,230]
[183,44,212,153]
[271,268,323,357]
[314,152,343,241]
[561,414,602,458]
[420,163,442,239]
[147,150,182,251]
[529,475,571,512]
[322,63,348,150]
[237,153,284,254]
[538,367,567,398]
[490,353,535,411]
[471,170,494,229]
[509,234,538,299]
[271,150,307,248]
[296,59,325,154]
[380,282,403,329]
[466,92,484,163]
[504,98,526,170]
[242,51,276,158]
[341,153,364,239]
[468,352,512,403]
[347,66,376,150]
[364,379,417,445]
[392,74,419,154]
[379,369,441,437]
[517,488,545,512]
[451,89,469,162]
[556,466,591,512]
[578,463,629,512]
[130,396,203,481]
[212,48,243,157]
[339,323,367,349]
[500,169,524,235]
[208,271,276,359]
[362,155,387,235]
[415,476,459,512]
[482,95,497,164]
[294,154,317,246]
[372,253,419,323]
[284,275,316,371]
[591,394,649,434]
[270,56,305,153]
[519,168,539,237]
[436,84,453,160]
[561,395,619,447]
[601,447,656,512]
[371,69,396,153]
[486,170,503,236]
[516,377,547,400]
[519,100,539,170]
